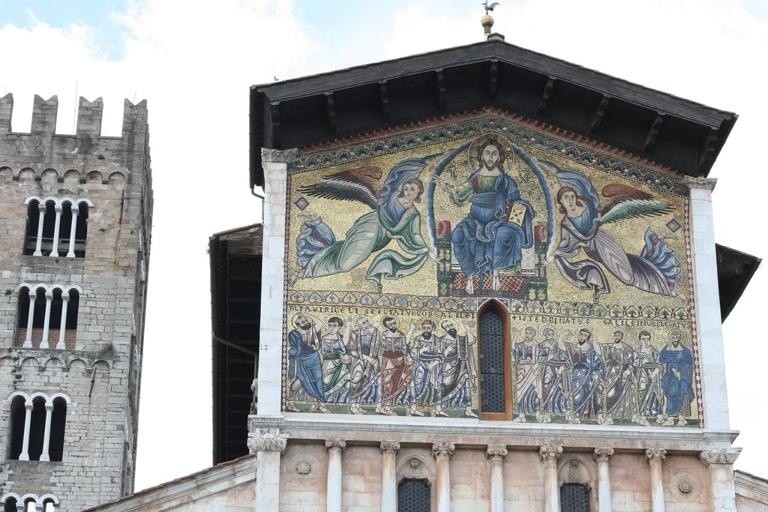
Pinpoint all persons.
[512,325,695,429]
[284,146,444,293]
[286,311,479,419]
[429,138,537,296]
[532,155,681,307]
